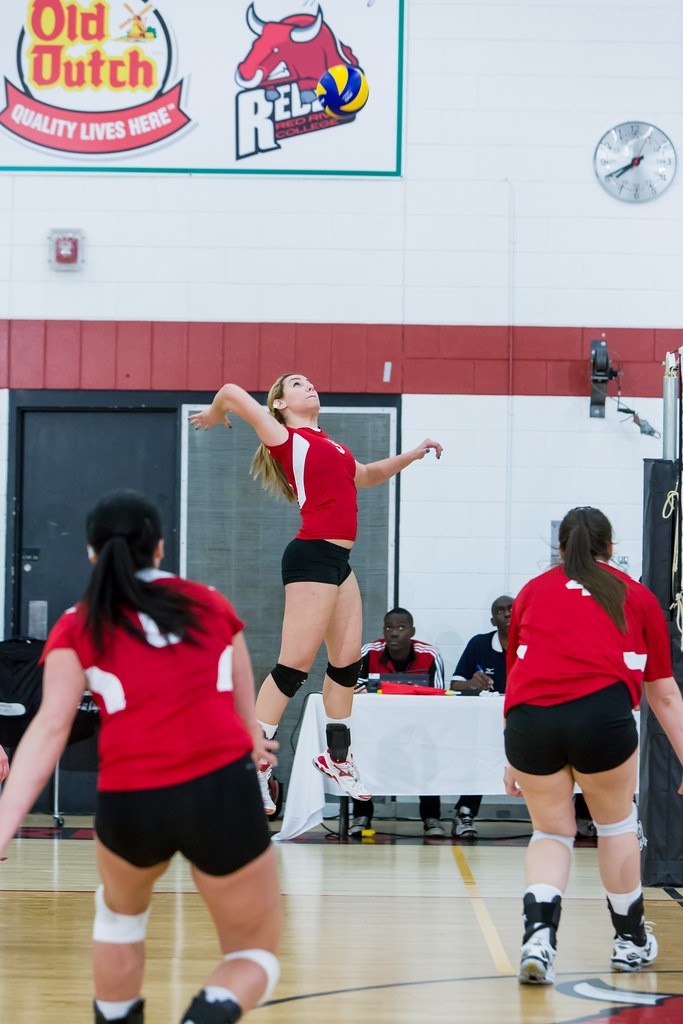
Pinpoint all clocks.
[591,122,679,206]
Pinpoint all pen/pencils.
[476,664,495,690]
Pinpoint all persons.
[449,596,596,840]
[0,490,288,1023]
[347,608,445,837]
[505,505,683,984]
[187,372,443,813]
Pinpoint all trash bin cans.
[0,636,98,816]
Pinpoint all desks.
[267,692,642,846]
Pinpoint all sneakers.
[610,921,657,971]
[517,939,556,984]
[256,764,277,815]
[575,819,594,837]
[312,749,371,802]
[350,816,369,835]
[450,813,477,838]
[423,819,447,836]
